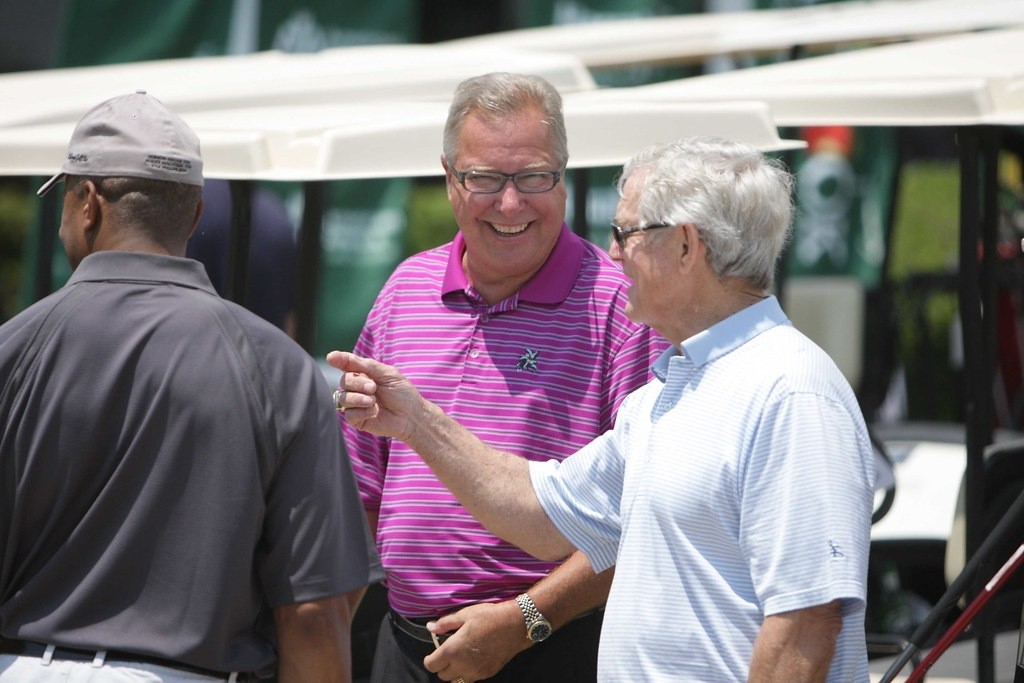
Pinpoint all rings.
[332,389,345,409]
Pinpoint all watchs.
[515,593,552,644]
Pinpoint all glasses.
[610,223,670,248]
[450,160,567,194]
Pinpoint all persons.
[336,73,673,683]
[326,137,873,683]
[0,92,387,681]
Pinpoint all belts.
[0,637,230,680]
[388,605,606,650]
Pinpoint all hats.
[38,90,205,198]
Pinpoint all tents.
[0,2,1024,184]
